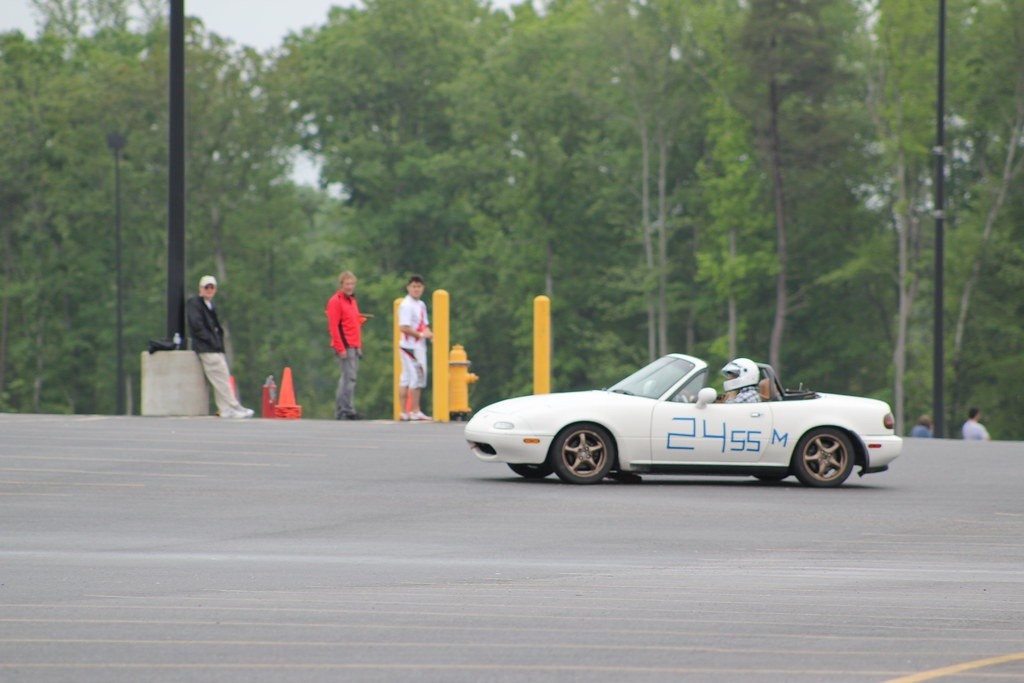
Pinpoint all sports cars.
[464,351,904,488]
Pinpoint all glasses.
[204,285,215,289]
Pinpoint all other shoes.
[219,407,255,420]
[401,411,432,421]
[336,412,367,420]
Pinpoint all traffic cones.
[274,364,303,420]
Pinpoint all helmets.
[720,357,761,392]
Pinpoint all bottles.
[173,333,181,349]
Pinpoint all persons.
[911,416,934,438]
[962,408,991,439]
[398,274,434,421]
[186,274,254,419]
[680,355,760,404]
[327,271,368,420]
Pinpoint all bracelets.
[690,395,695,400]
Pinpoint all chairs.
[757,363,781,401]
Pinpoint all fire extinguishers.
[263,375,277,418]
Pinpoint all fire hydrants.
[449,342,481,421]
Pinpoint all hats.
[198,274,218,287]
